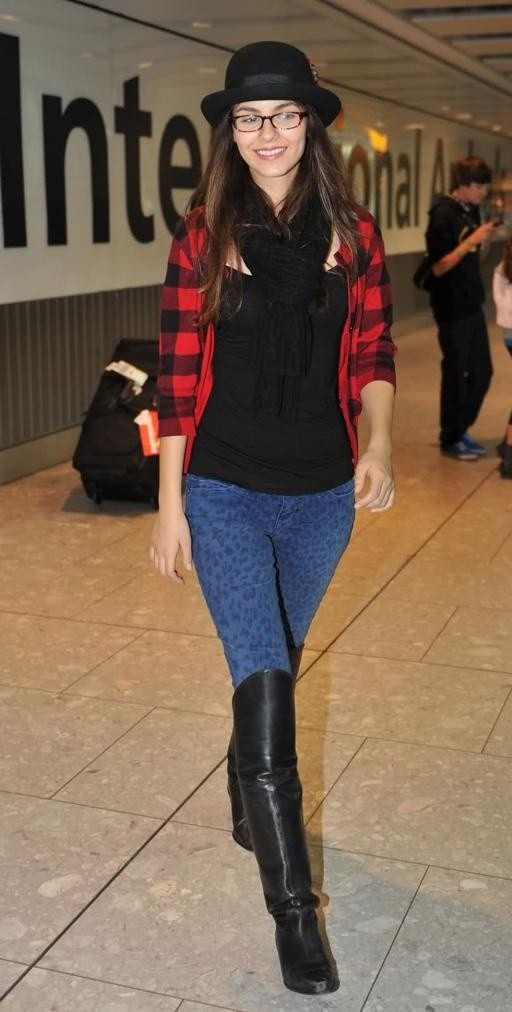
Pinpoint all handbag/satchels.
[412,256,440,292]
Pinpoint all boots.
[497,439,512,479]
[227,668,332,992]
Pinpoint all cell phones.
[493,220,504,227]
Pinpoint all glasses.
[232,111,303,130]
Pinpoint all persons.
[412,158,510,482]
[148,40,398,995]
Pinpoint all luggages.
[72,339,163,503]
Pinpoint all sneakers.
[440,431,486,460]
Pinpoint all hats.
[200,41,340,129]
